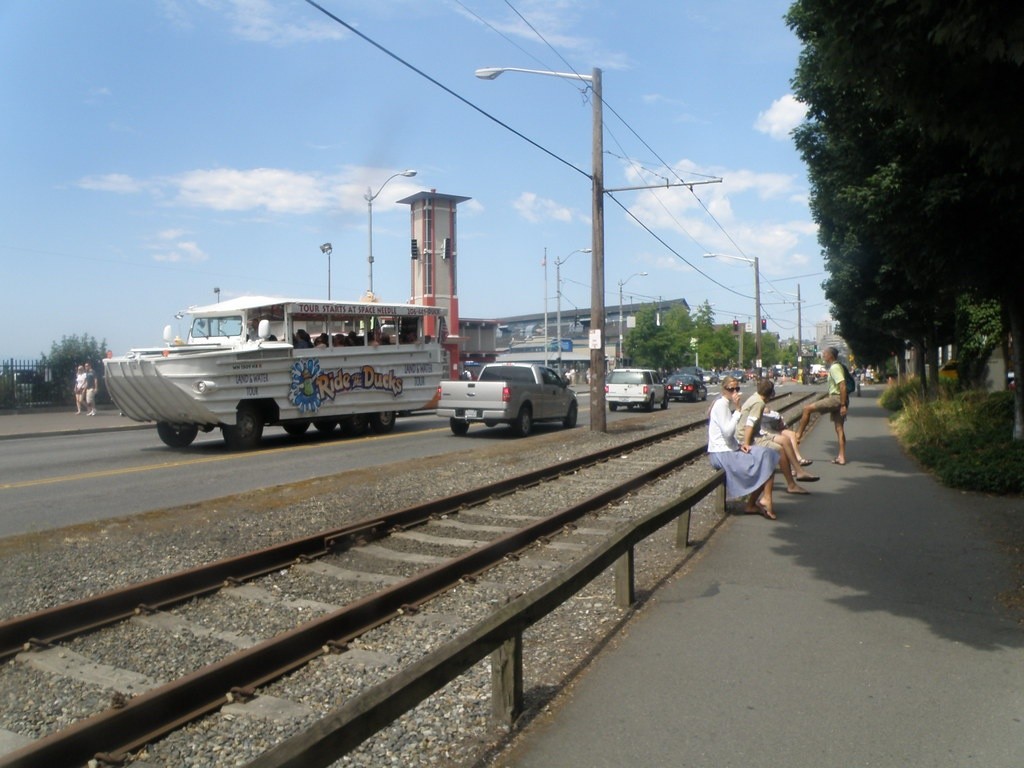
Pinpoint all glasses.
[725,386,740,392]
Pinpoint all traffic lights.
[762,319,767,330]
[814,345,817,353]
[733,320,738,331]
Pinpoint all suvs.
[679,367,703,381]
[605,368,670,412]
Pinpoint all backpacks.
[834,361,855,394]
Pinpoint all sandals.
[798,458,812,466]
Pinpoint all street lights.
[318,242,332,301]
[362,169,419,300]
[475,64,605,432]
[552,247,594,380]
[214,287,220,335]
[618,272,649,368]
[702,252,763,393]
[766,289,803,384]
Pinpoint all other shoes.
[87,408,96,416]
[74,412,81,415]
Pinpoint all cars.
[702,371,717,385]
[665,373,707,403]
[715,365,798,383]
[939,358,960,381]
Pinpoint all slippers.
[744,505,764,515]
[758,499,776,519]
[787,485,809,495]
[796,471,820,482]
[832,457,844,465]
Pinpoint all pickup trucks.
[436,362,577,438]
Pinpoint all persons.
[769,366,788,379]
[399,329,417,343]
[707,377,780,520]
[425,335,431,344]
[761,390,813,476]
[368,331,390,345]
[75,363,98,415]
[710,367,722,374]
[565,368,576,385]
[269,335,277,341]
[797,347,849,465]
[586,368,591,384]
[850,364,874,383]
[736,380,820,494]
[332,331,365,347]
[293,329,329,349]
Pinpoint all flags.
[441,317,449,342]
